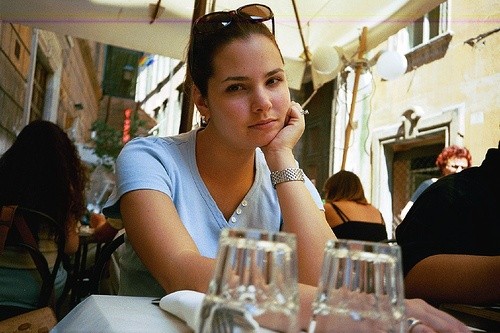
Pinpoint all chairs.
[0,206,125,321]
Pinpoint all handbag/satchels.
[0,306,57,333]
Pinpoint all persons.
[89,210,127,295]
[395,143,500,303]
[0,119,79,320]
[115,4,472,333]
[400,146,473,222]
[324,170,388,292]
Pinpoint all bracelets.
[270,168,305,190]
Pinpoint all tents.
[0,0,448,171]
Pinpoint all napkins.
[159,289,259,333]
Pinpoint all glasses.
[190,4,275,49]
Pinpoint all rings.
[406,316,421,333]
[300,109,309,115]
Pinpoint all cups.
[194,228,303,333]
[306,239,407,333]
